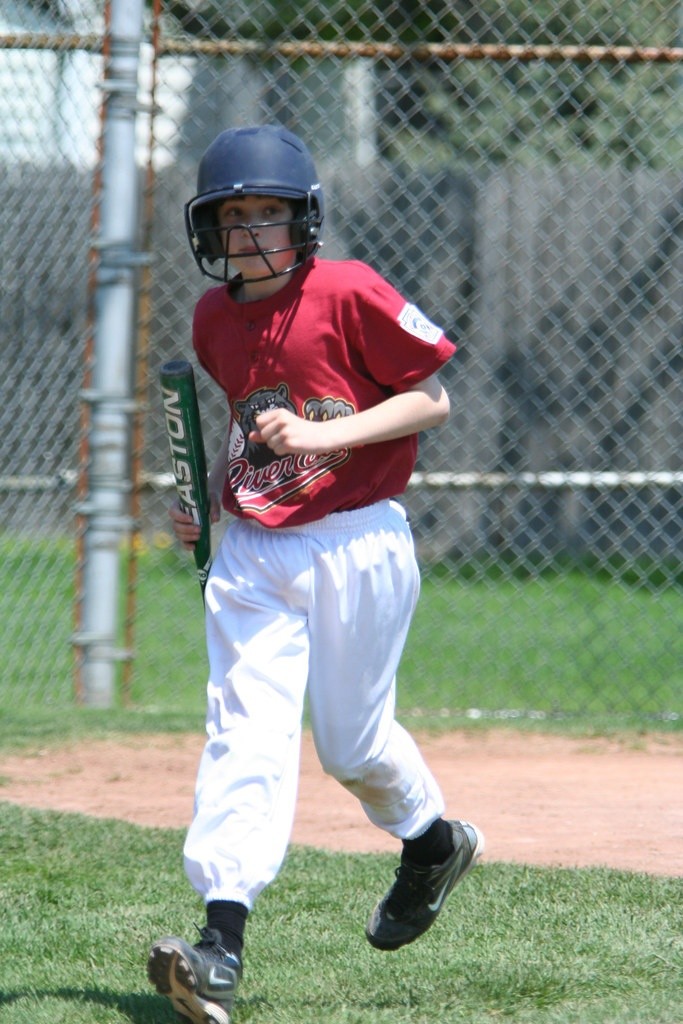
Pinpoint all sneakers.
[146,922,243,1024]
[366,819,485,951]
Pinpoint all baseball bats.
[158,361,215,613]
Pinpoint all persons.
[147,125,483,1024]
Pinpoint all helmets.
[191,125,324,265]
